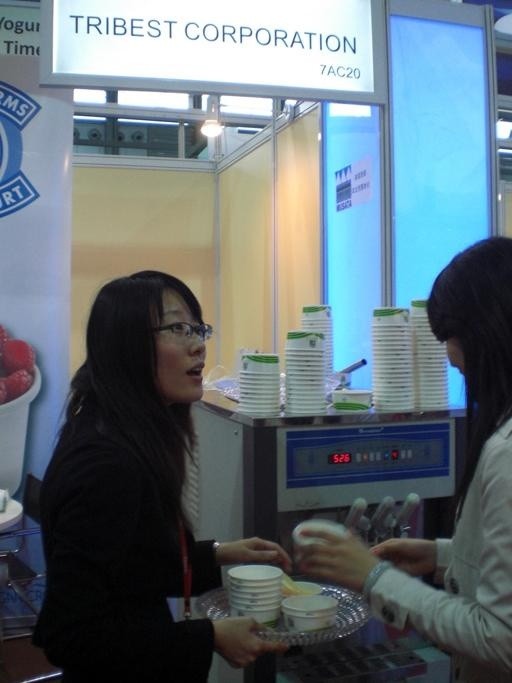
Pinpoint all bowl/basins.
[235,293,444,415]
[1,361,42,497]
[223,567,338,631]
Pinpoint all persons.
[30,267,295,683]
[297,233,512,683]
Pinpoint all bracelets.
[212,540,221,551]
[361,559,392,601]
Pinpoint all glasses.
[151,321,213,342]
